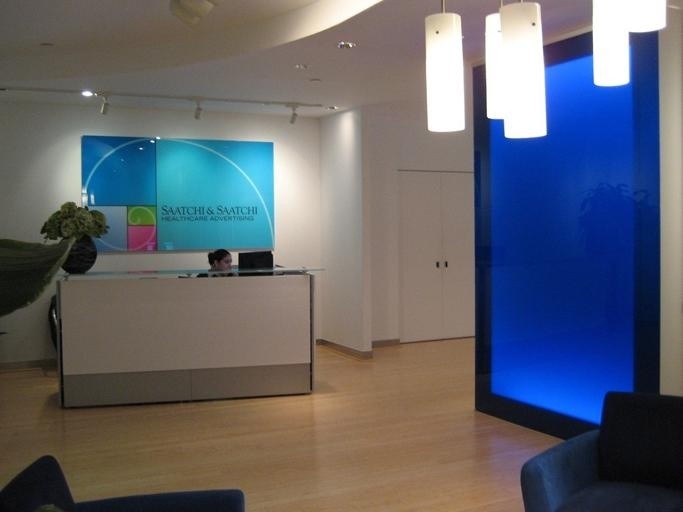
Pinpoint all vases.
[59,233,98,274]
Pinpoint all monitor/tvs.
[238,251,273,269]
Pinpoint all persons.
[196,248,235,278]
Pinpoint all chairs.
[520,391,683,512]
[0,455,246,512]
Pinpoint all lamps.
[191,97,202,120]
[287,104,298,124]
[424,0,667,139]
[94,92,111,115]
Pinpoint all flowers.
[40,201,110,242]
